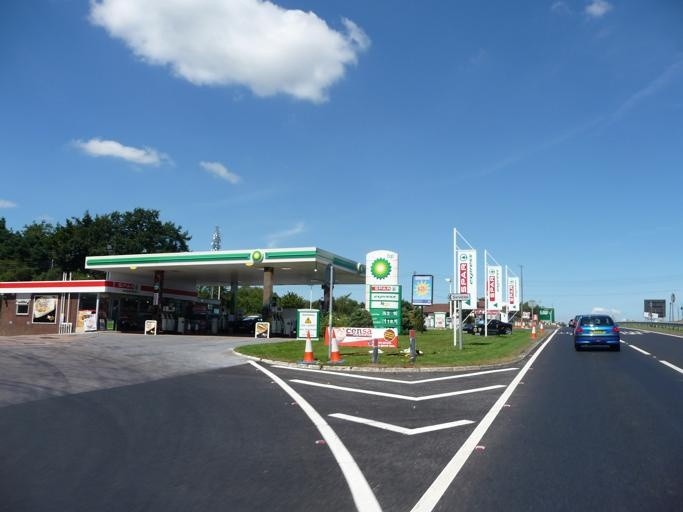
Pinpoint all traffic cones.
[327,329,345,364]
[539,323,544,332]
[300,330,316,364]
[532,325,536,338]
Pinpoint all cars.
[569,315,620,351]
[238,315,262,334]
[463,319,512,334]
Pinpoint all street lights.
[445,278,452,329]
